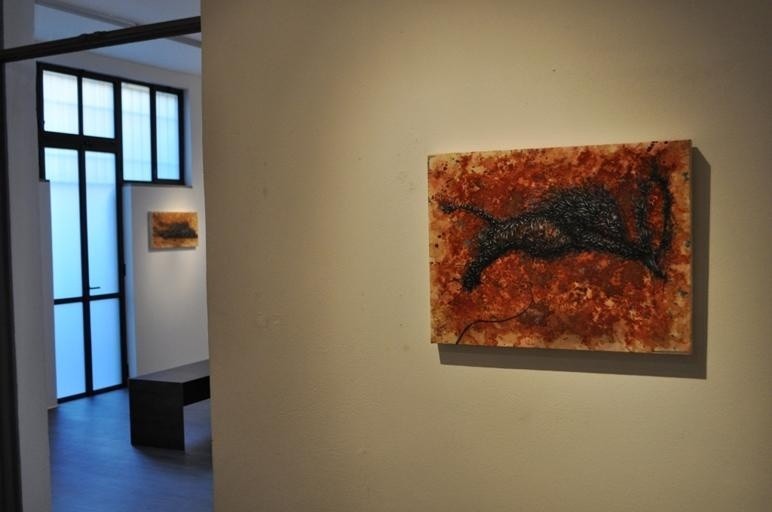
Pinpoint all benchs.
[126,356,210,454]
